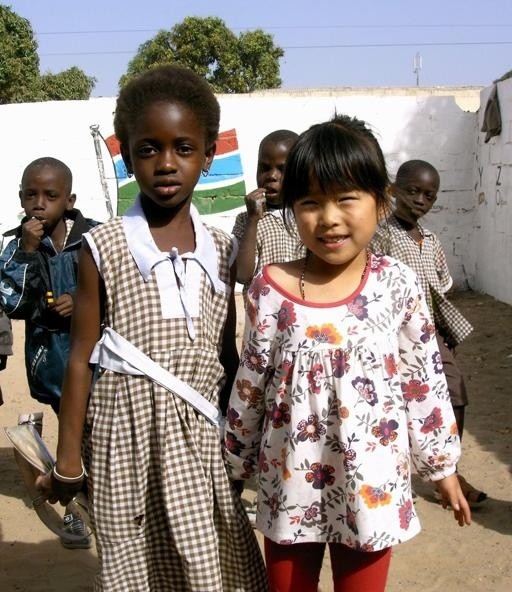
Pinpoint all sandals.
[431,474,491,508]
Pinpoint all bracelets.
[52,457,89,483]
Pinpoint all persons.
[34,67,267,592]
[1,156,107,549]
[367,159,489,507]
[224,116,472,592]
[231,130,309,530]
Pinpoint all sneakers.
[60,499,93,550]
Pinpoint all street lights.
[412,51,423,87]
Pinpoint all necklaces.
[300,248,371,302]
